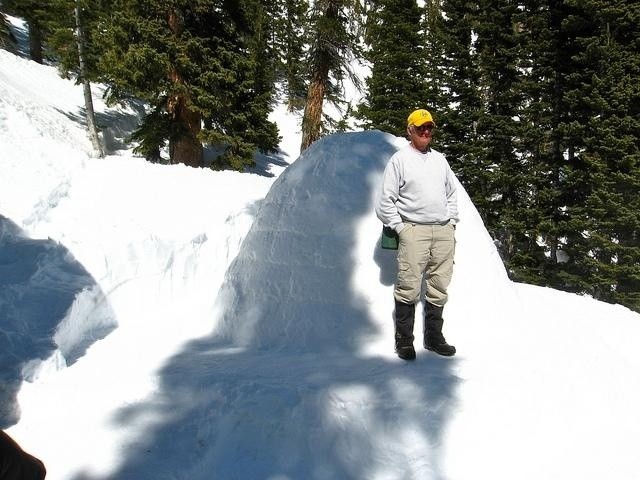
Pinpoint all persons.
[375,109,460,360]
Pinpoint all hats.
[407,109,436,128]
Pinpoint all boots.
[394,297,417,361]
[422,300,457,357]
